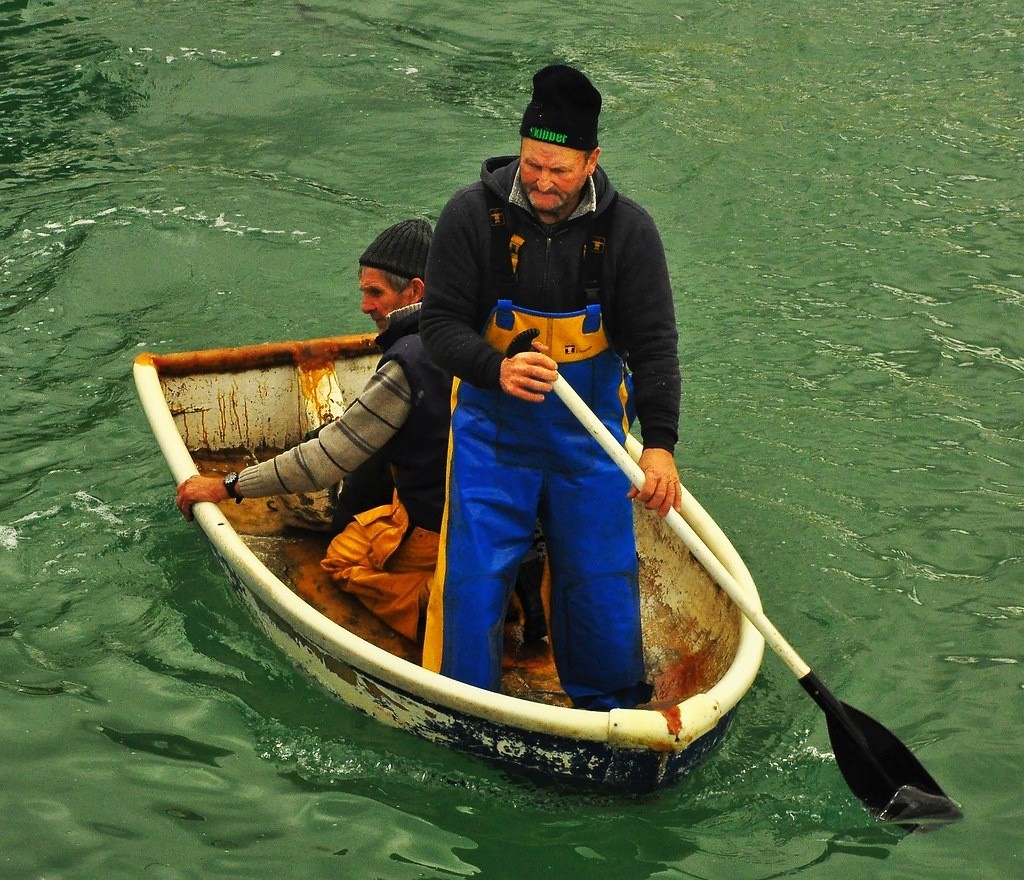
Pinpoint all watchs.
[223,471,244,504]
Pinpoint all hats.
[519,64,602,151]
[359,219,433,283]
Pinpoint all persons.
[177,219,550,662]
[420,65,682,710]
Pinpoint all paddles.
[504,327,967,832]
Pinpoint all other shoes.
[510,554,547,643]
[420,575,434,605]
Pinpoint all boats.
[130,335,765,797]
